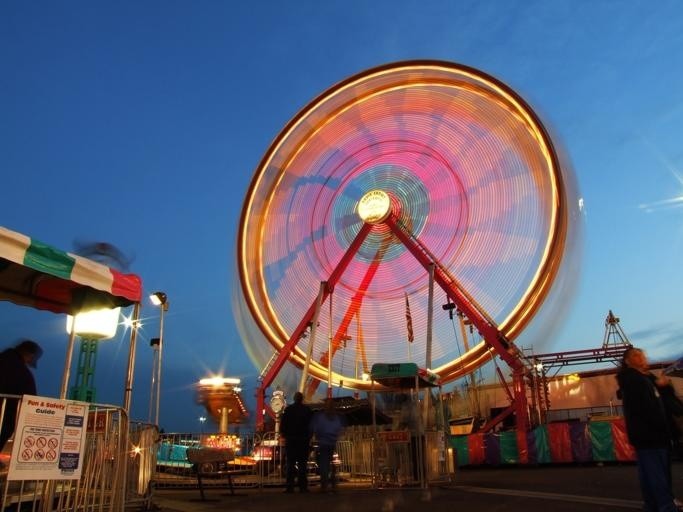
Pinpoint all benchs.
[185,447,252,501]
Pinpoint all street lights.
[149,292,167,426]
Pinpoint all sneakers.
[282,487,310,493]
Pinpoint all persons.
[0,340,43,454]
[615,346,682,511]
[279,392,314,494]
[309,397,342,494]
[242,438,252,456]
